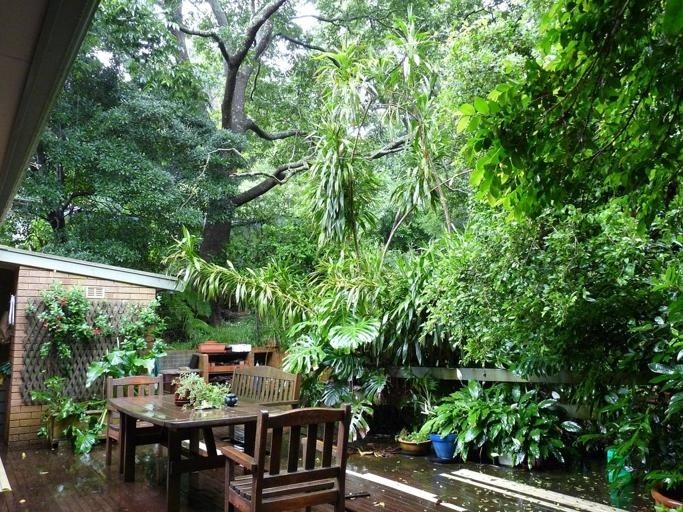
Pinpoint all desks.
[108,394,288,512]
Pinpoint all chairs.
[221,403,352,512]
[105,373,164,473]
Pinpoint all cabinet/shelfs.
[191,348,278,385]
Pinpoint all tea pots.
[223,393,238,405]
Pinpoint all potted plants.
[627,258,683,512]
[420,404,467,460]
[170,370,224,411]
[397,431,432,456]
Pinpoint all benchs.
[229,364,301,459]
